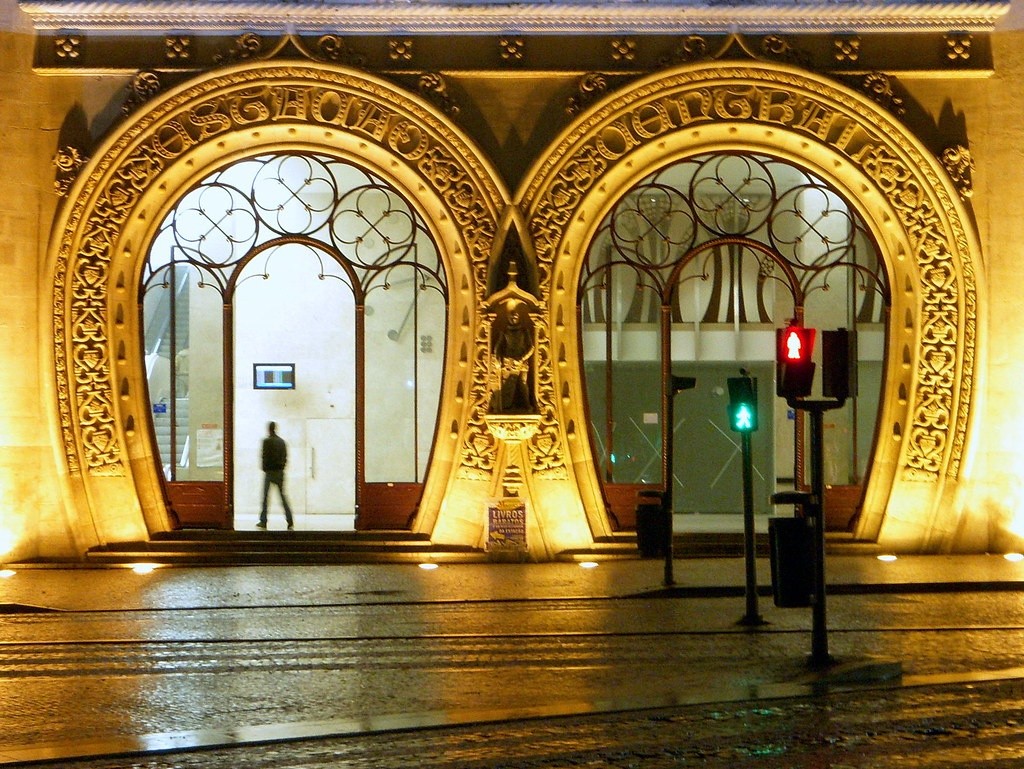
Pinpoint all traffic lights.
[822,328,859,398]
[668,375,696,395]
[726,376,759,434]
[777,327,817,396]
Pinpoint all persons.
[175,344,189,397]
[256,422,294,530]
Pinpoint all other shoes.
[287,523,294,530]
[256,521,266,528]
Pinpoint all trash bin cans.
[767,490,814,609]
[635,490,668,560]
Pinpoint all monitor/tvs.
[253,363,296,390]
